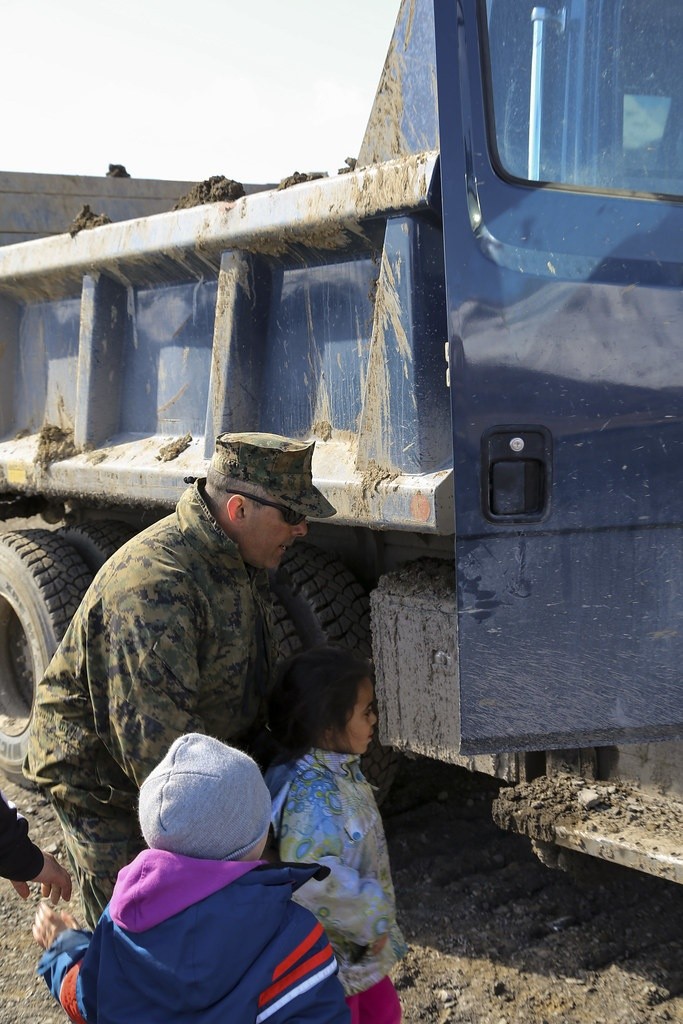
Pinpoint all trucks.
[1,0,683,883]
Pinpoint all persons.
[31,425,337,937]
[263,654,409,1024]
[28,731,352,1024]
[0,788,75,905]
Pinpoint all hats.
[138,732,271,867]
[212,433,337,518]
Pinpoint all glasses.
[226,489,307,524]
